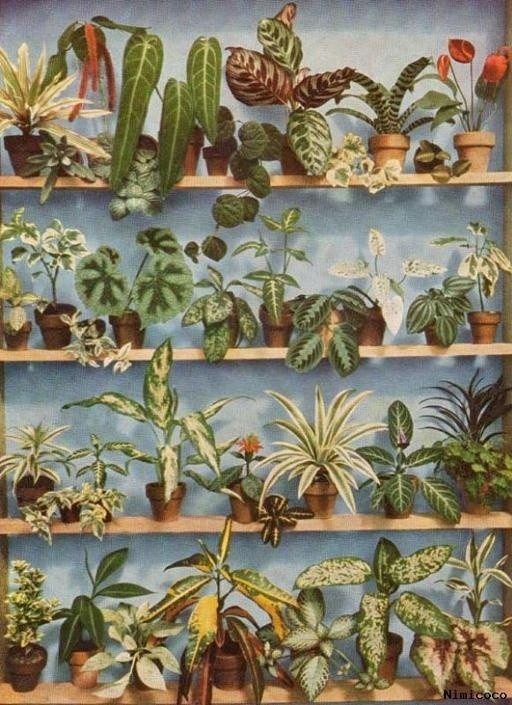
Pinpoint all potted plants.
[0,2,512,704]
